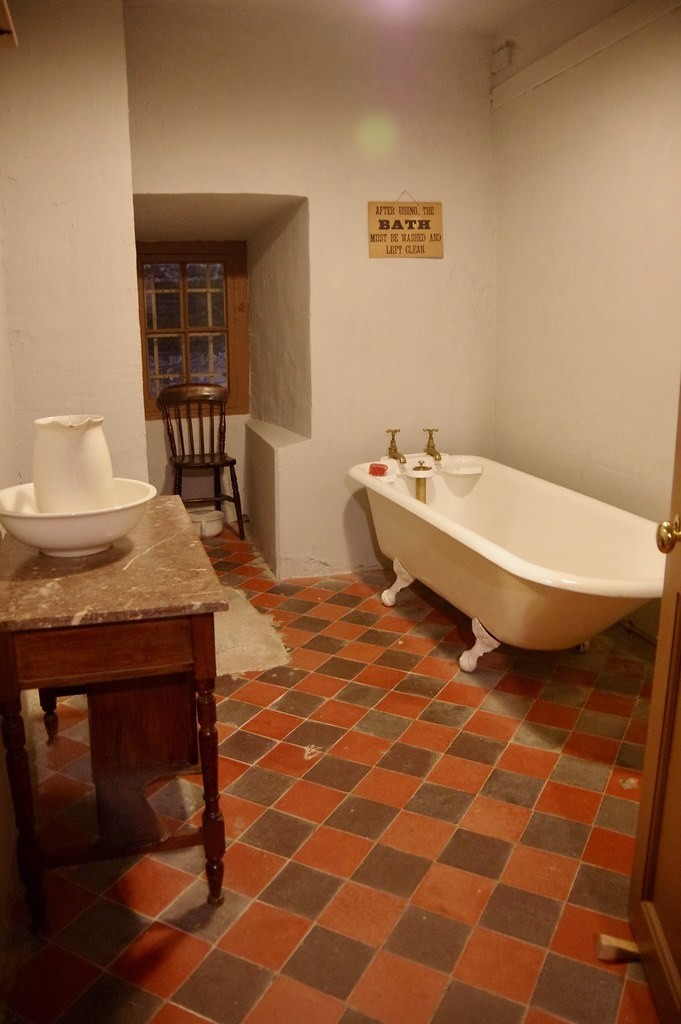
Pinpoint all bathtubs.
[347,453,667,653]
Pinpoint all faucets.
[421,428,442,461]
[385,428,409,464]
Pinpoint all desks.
[0,494,228,945]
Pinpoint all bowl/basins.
[0,475,156,558]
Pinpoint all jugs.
[32,414,112,513]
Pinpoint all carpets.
[211,586,293,675]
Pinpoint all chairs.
[157,383,245,540]
